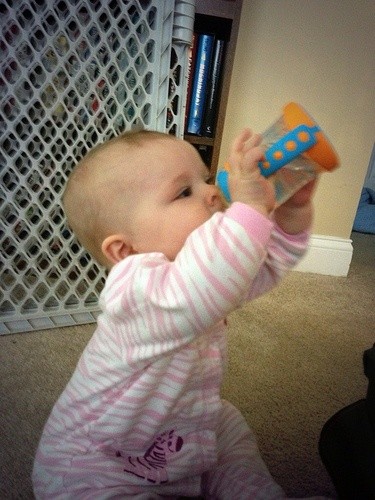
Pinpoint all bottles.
[215,99,341,222]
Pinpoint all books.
[0,4,228,272]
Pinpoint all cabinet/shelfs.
[169,1,242,188]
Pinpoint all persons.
[30,127,322,500]
